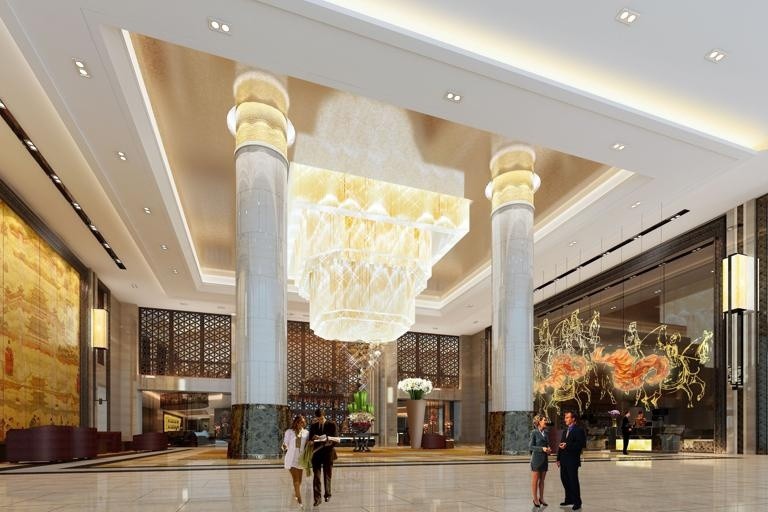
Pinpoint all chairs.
[6,426,121,464]
[423,432,445,449]
[133,432,168,452]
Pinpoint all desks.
[343,433,380,451]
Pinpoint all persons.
[529,414,552,507]
[631,411,647,438]
[622,411,633,455]
[281,414,310,511]
[307,408,339,506]
[215,415,232,439]
[556,410,585,511]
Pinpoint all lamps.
[747,256,760,390]
[722,253,747,390]
[93,308,109,401]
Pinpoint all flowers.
[346,410,376,424]
[398,378,433,400]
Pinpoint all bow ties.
[566,426,573,432]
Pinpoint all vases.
[351,424,371,433]
[407,400,426,449]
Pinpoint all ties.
[320,424,322,433]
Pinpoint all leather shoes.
[533,500,540,508]
[539,499,548,506]
[313,500,321,506]
[623,452,629,455]
[325,496,329,502]
[572,504,581,510]
[560,502,573,505]
